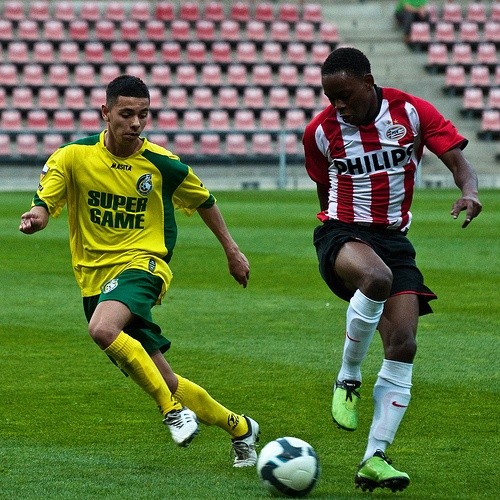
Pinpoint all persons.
[301,47,483,496]
[393,0,437,44]
[19,75,259,468]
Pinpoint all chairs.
[1,0,500,161]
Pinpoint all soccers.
[257,436,320,498]
